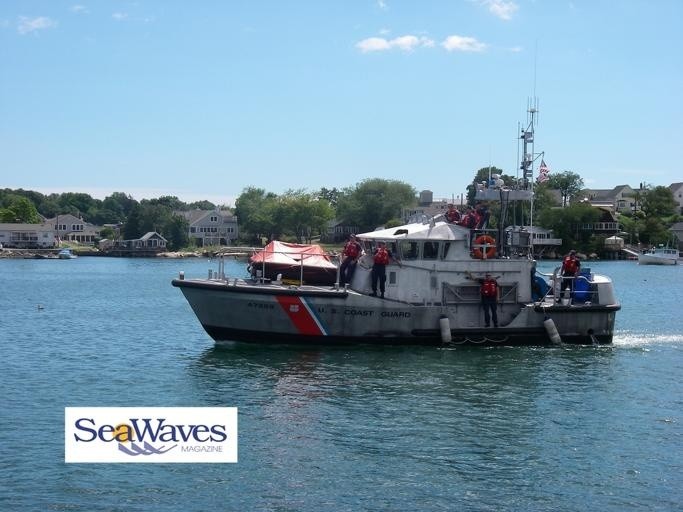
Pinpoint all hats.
[448,204,453,207]
[350,234,356,238]
[569,250,576,255]
[378,239,385,244]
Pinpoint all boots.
[369,291,384,298]
[571,298,574,305]
[556,298,561,303]
[485,322,498,327]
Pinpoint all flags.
[538,159,550,183]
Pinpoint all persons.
[340,233,362,286]
[370,242,402,298]
[445,202,490,229]
[556,249,580,303]
[465,270,500,327]
[651,246,656,254]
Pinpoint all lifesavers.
[474,235,496,259]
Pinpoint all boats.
[170,173,622,349]
[58,250,78,259]
[638,248,680,266]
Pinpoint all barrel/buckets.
[574,268,591,300]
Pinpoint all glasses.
[486,275,491,276]
